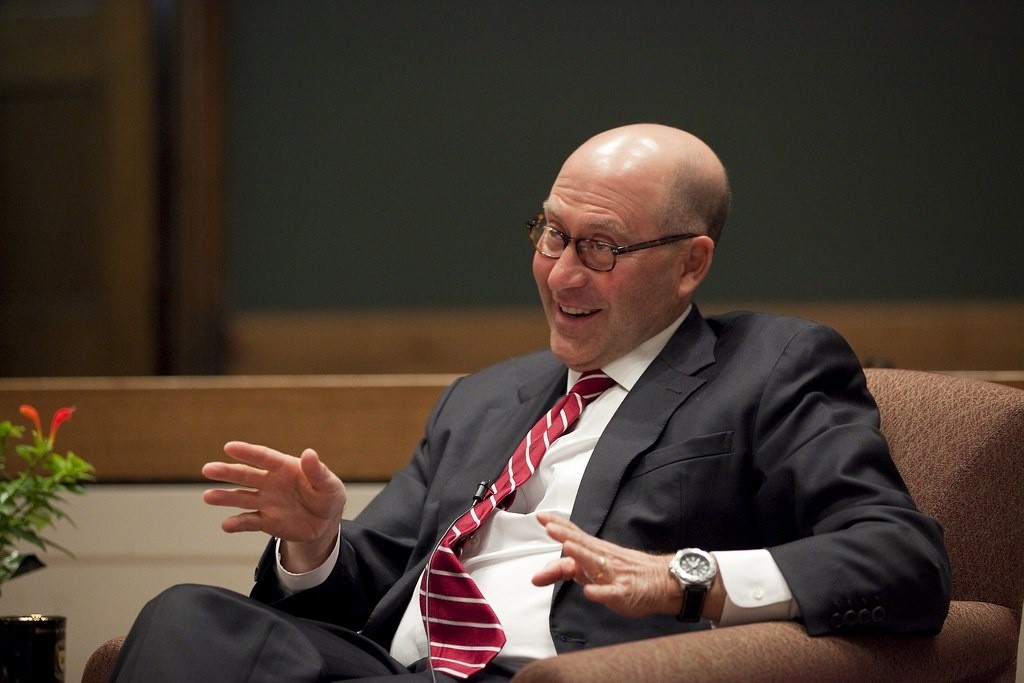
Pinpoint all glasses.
[526,214,698,272]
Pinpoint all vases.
[0,617,66,683]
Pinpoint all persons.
[105,122,954,683]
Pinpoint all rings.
[585,553,607,581]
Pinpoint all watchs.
[668,547,717,625]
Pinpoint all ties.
[419,368,618,680]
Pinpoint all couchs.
[84,369,1024,683]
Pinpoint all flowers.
[0,398,95,585]
